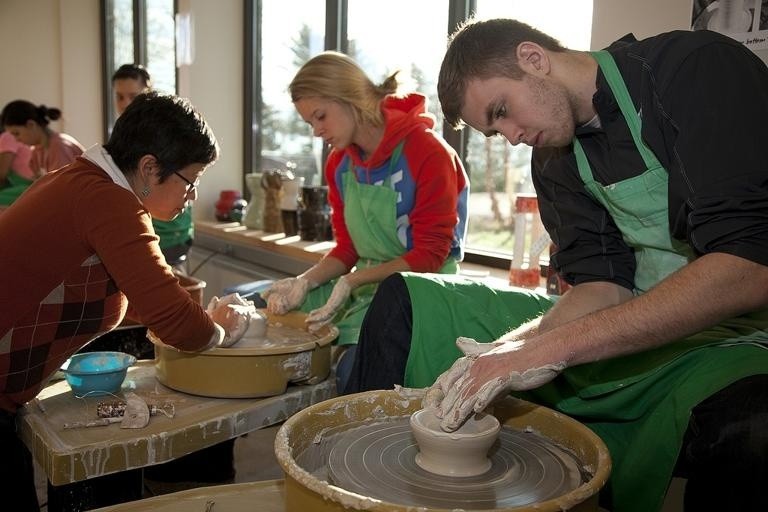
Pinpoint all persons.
[4,100,84,177]
[344,18,768,511]
[240,51,469,394]
[103,65,193,327]
[2,123,28,182]
[2,92,253,512]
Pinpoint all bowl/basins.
[61,349,138,399]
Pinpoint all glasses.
[167,165,203,196]
